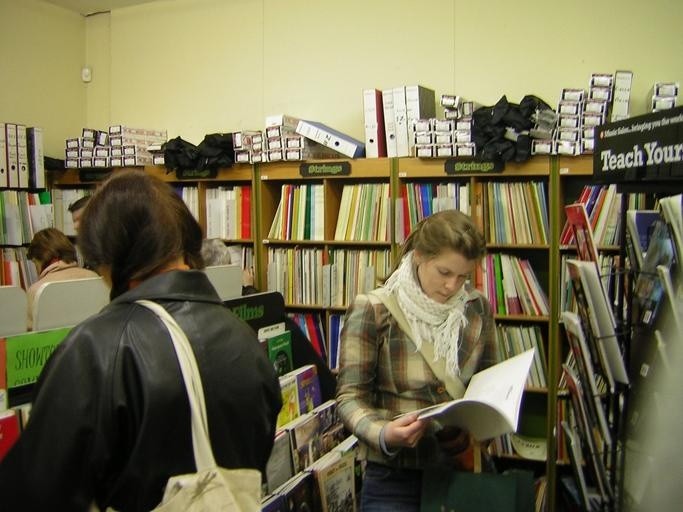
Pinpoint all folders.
[0,122,45,189]
[295,84,436,159]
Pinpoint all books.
[1,403,32,459]
[489,324,548,511]
[268,181,549,316]
[395,348,536,442]
[1,187,97,288]
[182,184,254,268]
[557,185,683,512]
[257,314,363,512]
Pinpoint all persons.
[26,196,98,330]
[0,170,282,512]
[204,238,258,295]
[334,210,499,512]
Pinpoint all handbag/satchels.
[164,132,234,179]
[472,95,552,163]
[150,467,262,512]
[421,463,534,512]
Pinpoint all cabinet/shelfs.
[0,154,674,512]
